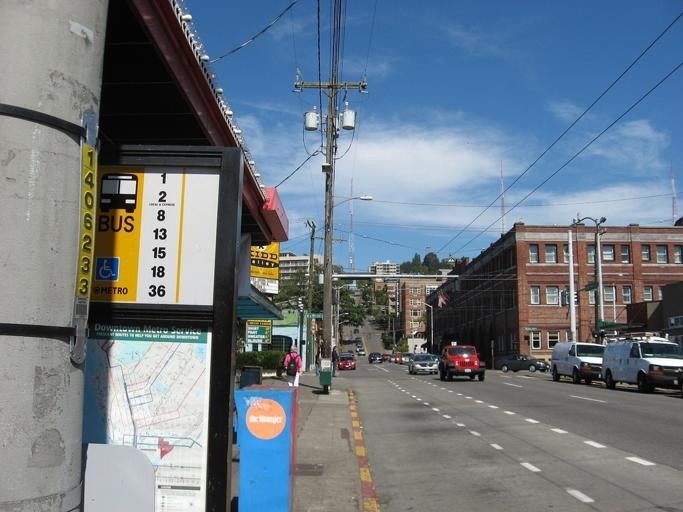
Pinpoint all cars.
[496,352,549,373]
[424,352,439,367]
[352,326,366,357]
[336,348,356,370]
[407,354,438,376]
[367,350,412,366]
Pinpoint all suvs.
[437,346,486,382]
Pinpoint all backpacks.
[287,353,299,376]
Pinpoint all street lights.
[328,192,374,211]
[566,213,606,330]
[413,300,433,353]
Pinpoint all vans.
[549,342,608,384]
[600,333,682,395]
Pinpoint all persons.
[332,346,339,377]
[284,345,302,387]
[315,351,321,376]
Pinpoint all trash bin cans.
[240,365,263,388]
